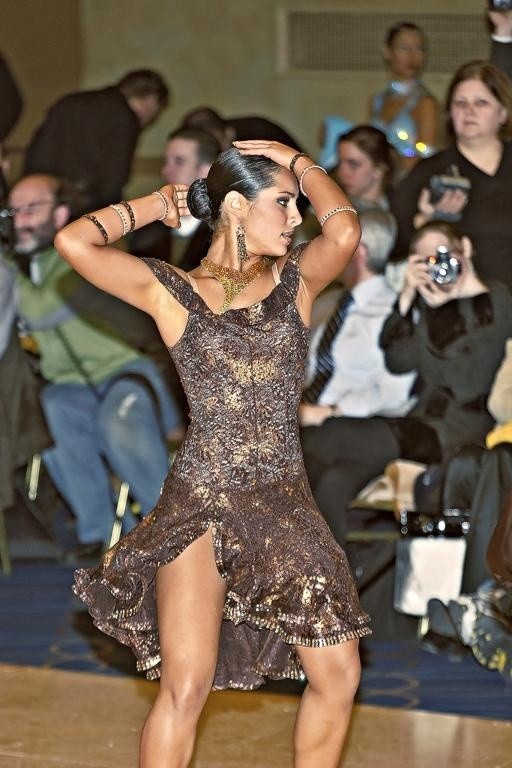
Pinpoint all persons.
[3,108,512,689]
[487,0,512,74]
[50,136,380,765]
[24,70,166,199]
[320,24,437,189]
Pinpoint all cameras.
[428,161,474,205]
[422,244,462,290]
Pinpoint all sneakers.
[426,590,511,685]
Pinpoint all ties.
[298,289,356,408]
